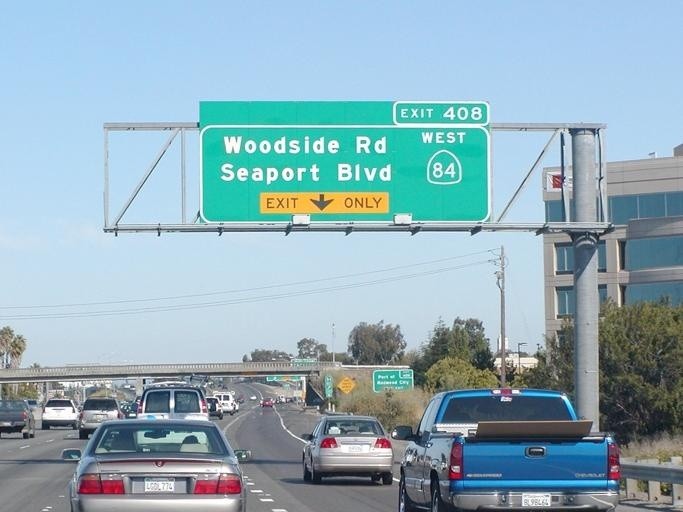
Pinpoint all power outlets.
[390,385,621,512]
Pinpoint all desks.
[517,342,528,374]
[330,322,336,361]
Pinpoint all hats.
[53,417,255,512]
[39,383,300,423]
[298,412,394,486]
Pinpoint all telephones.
[290,358,318,367]
[371,369,416,394]
[265,376,301,383]
[323,375,333,400]
[390,99,493,128]
[197,123,492,231]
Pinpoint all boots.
[39,398,79,431]
[73,395,125,441]
[24,399,36,409]
[0,399,36,439]
